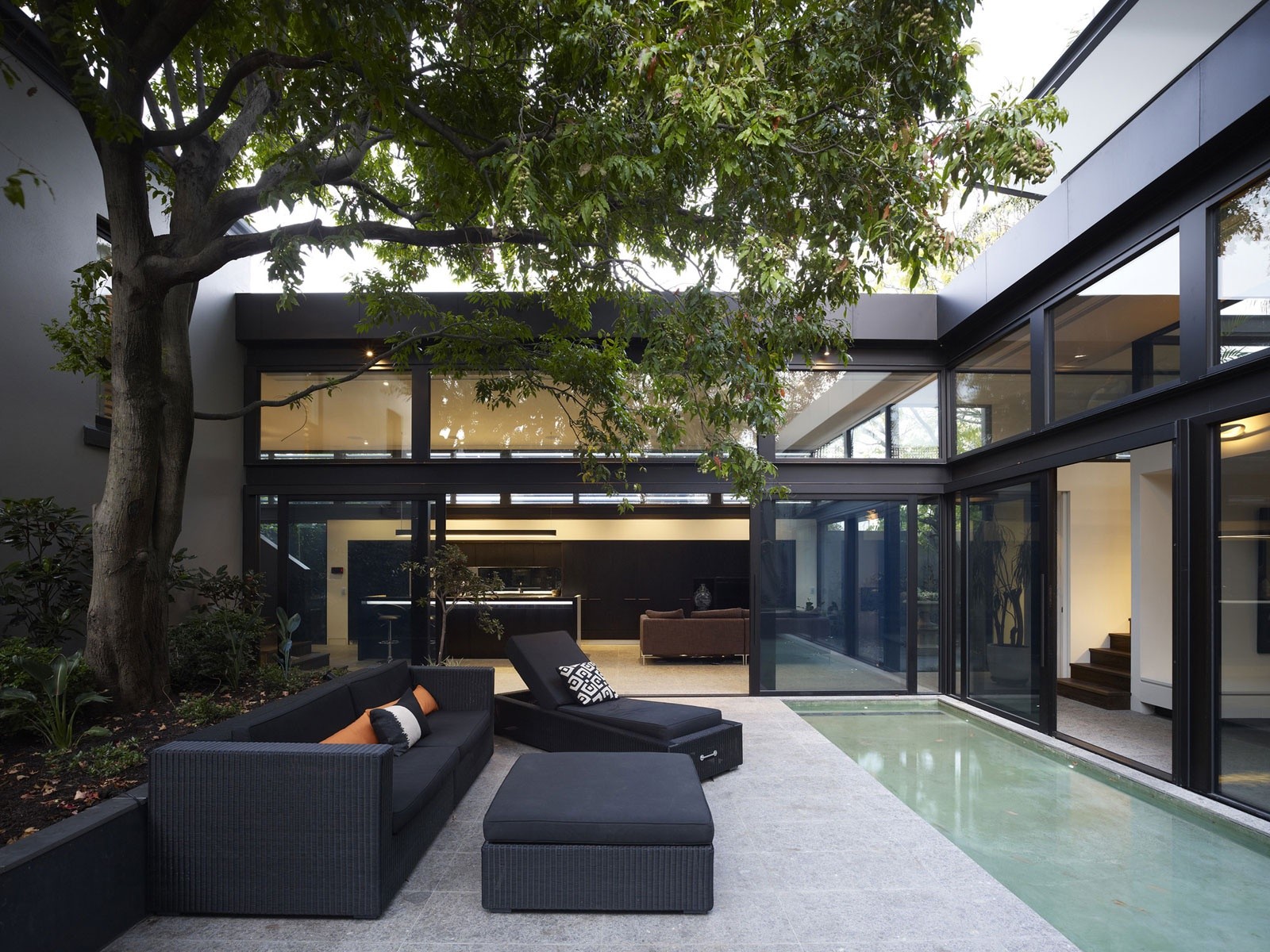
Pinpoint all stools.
[373,604,408,665]
[478,753,715,916]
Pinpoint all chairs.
[494,629,743,781]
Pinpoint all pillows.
[366,683,439,720]
[645,608,685,619]
[318,711,380,744]
[369,687,432,756]
[555,661,620,707]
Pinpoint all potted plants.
[978,517,1035,690]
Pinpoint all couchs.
[148,659,497,919]
[638,609,753,666]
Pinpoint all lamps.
[1220,423,1246,441]
